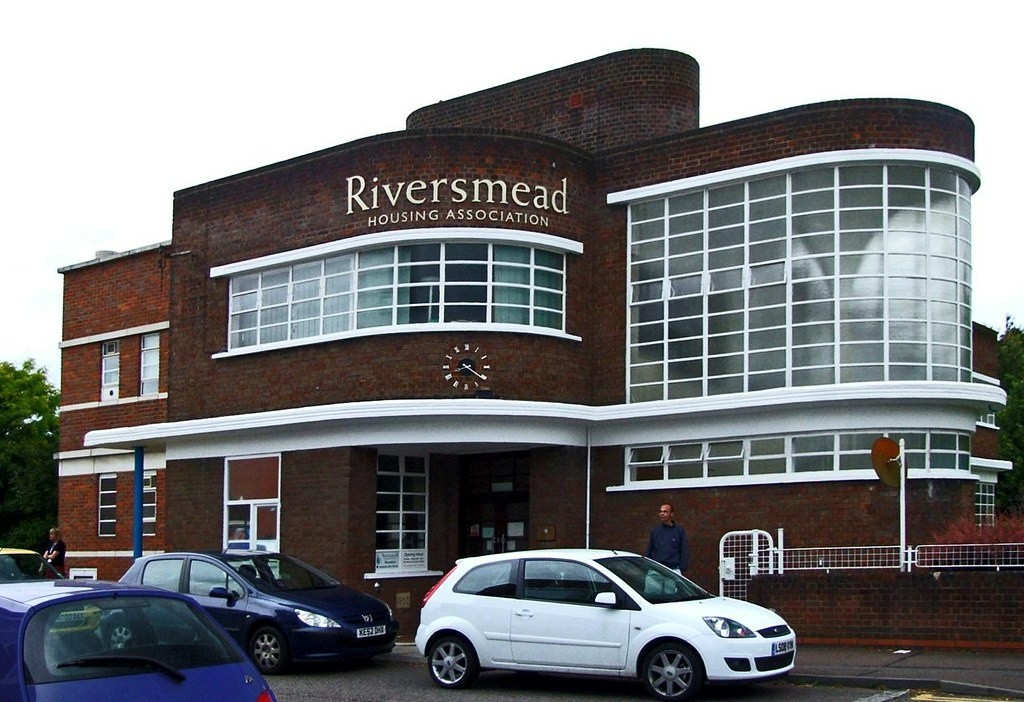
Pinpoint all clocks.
[443,344,491,390]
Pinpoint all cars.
[99,552,399,662]
[0,579,280,702]
[413,550,796,701]
[0,545,63,585]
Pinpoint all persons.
[642,503,689,596]
[38,528,66,579]
